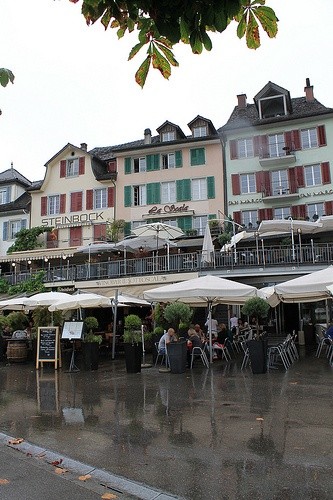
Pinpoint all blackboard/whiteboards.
[36,326,58,362]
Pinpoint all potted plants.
[6,312,30,363]
[83,317,102,372]
[162,302,193,373]
[241,297,268,374]
[122,315,143,374]
[144,327,165,352]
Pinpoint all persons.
[194,324,205,342]
[230,314,239,336]
[186,328,202,367]
[204,314,220,339]
[158,328,177,362]
[237,318,251,339]
[215,323,228,345]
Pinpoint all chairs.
[226,334,241,354]
[315,329,332,358]
[155,342,168,368]
[212,338,231,361]
[329,340,333,367]
[241,332,298,370]
[190,343,209,369]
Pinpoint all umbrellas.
[48,292,115,342]
[76,215,333,267]
[142,274,261,365]
[258,264,333,342]
[109,295,151,306]
[22,290,75,326]
[0,298,30,312]
